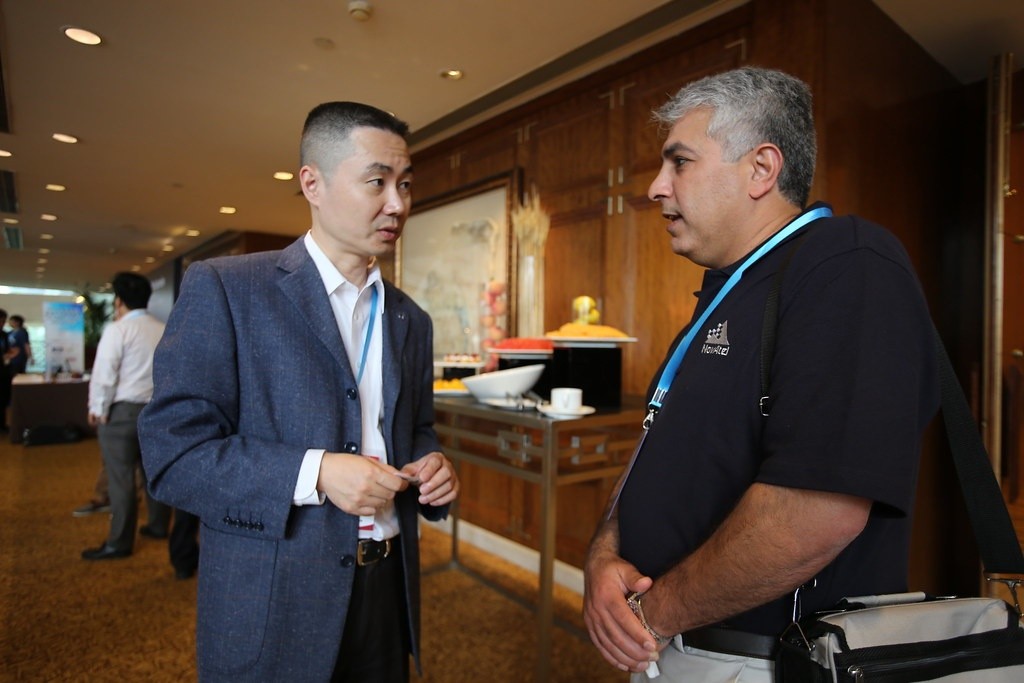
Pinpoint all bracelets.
[626,594,666,647]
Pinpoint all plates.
[433,390,470,396]
[432,362,485,367]
[484,399,536,410]
[539,406,595,420]
[550,337,638,347]
[487,350,553,358]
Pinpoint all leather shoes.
[81,543,132,560]
[139,526,168,540]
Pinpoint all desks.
[429,395,645,683]
[11,374,92,442]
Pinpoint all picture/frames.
[390,164,524,372]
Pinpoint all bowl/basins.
[462,365,544,402]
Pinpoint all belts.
[355,534,403,566]
[680,626,774,658]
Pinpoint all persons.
[138,103,460,683]
[0,269,199,582]
[580,65,941,683]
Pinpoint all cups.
[551,389,582,414]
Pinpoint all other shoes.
[75,497,110,516]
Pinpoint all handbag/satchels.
[775,591,1024,683]
